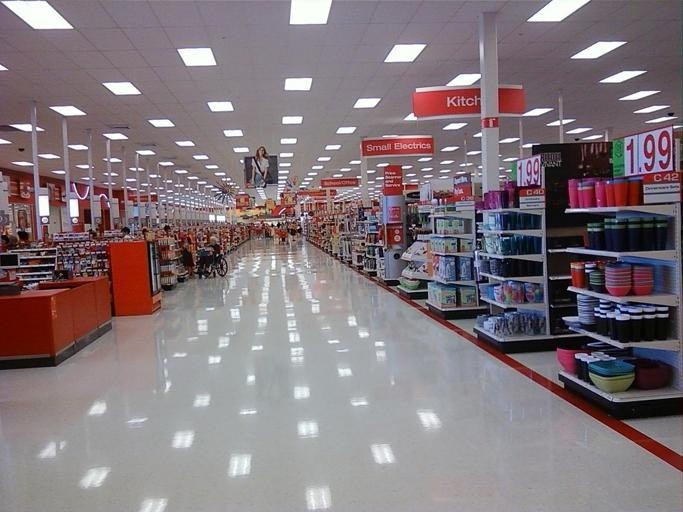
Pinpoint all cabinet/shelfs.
[0,224,251,368]
[300,175,681,418]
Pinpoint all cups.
[569,261,606,291]
[574,352,617,386]
[586,215,668,253]
[594,298,670,343]
[568,176,642,209]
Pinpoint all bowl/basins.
[576,294,600,332]
[589,262,655,297]
[557,344,593,374]
[589,360,635,393]
[625,360,670,389]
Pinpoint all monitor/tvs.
[0,253,20,270]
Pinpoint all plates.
[561,315,579,323]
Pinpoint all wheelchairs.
[193,248,228,279]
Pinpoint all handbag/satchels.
[262,172,273,184]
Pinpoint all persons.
[179,236,196,277]
[163,224,173,236]
[0,233,9,255]
[198,235,221,274]
[140,228,154,240]
[42,232,51,242]
[7,232,18,251]
[249,145,271,189]
[16,229,31,248]
[268,221,303,241]
[119,226,135,239]
